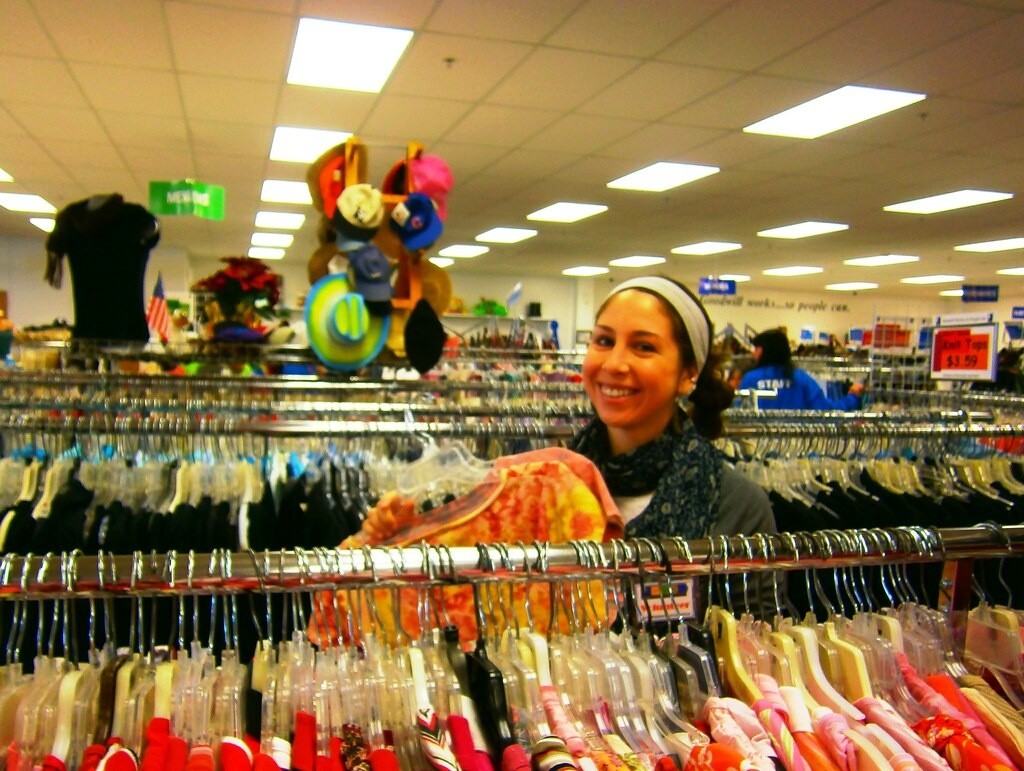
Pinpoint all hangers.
[0,348,1024,771]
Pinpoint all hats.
[305,142,454,375]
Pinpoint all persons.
[735,329,864,410]
[968,346,1024,396]
[45,193,160,344]
[514,274,795,638]
